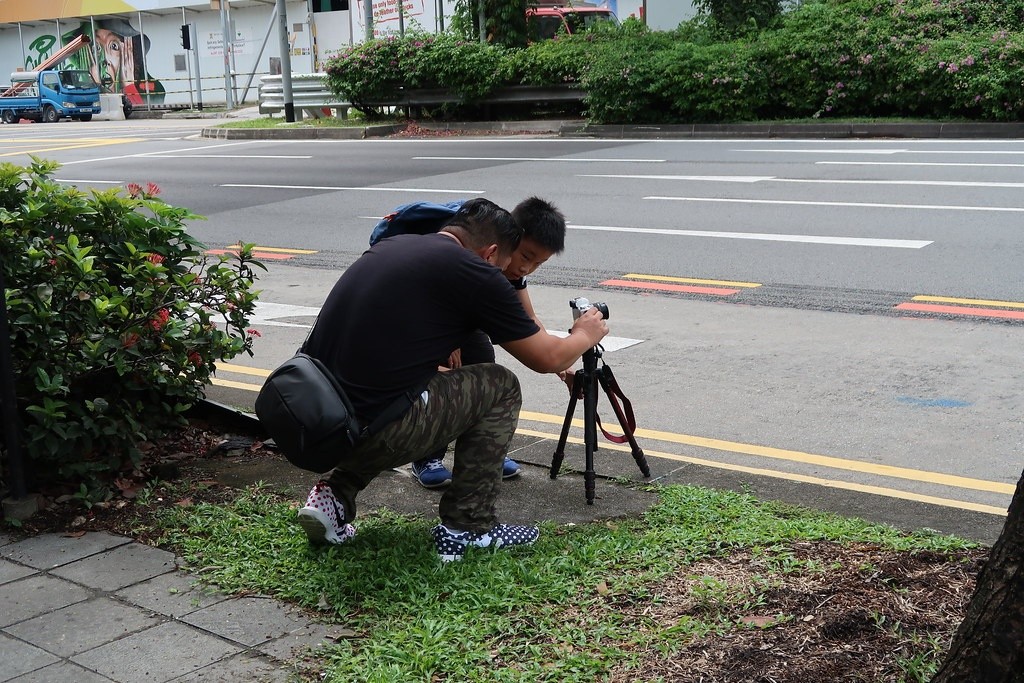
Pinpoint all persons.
[296,198,610,562]
[369,196,584,489]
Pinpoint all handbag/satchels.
[255,353,363,473]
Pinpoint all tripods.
[550,328,651,506]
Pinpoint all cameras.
[570,297,609,321]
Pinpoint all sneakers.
[429,524,539,563]
[411,459,452,488]
[297,483,354,545]
[501,456,521,478]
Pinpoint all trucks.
[486,0,621,47]
[1,70,102,122]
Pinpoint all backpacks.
[369,200,466,246]
